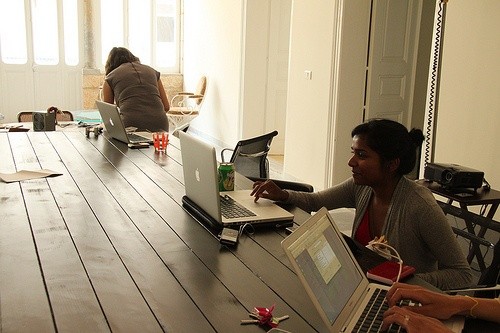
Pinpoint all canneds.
[217,163,235,191]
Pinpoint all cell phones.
[128,142,150,148]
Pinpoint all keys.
[240,304,293,330]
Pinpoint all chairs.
[166,77,206,136]
[222,131,314,194]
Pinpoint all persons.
[250,118,475,290]
[100,46,171,131]
[380,280,500,333]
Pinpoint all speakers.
[32,112,57,132]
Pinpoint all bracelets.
[465,293,479,319]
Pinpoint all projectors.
[424,162,484,189]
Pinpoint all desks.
[0,122,434,333]
[415,179,500,276]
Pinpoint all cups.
[152,133,169,151]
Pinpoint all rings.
[401,312,409,329]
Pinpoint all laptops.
[178,130,294,233]
[283,211,423,333]
[96,100,162,144]
